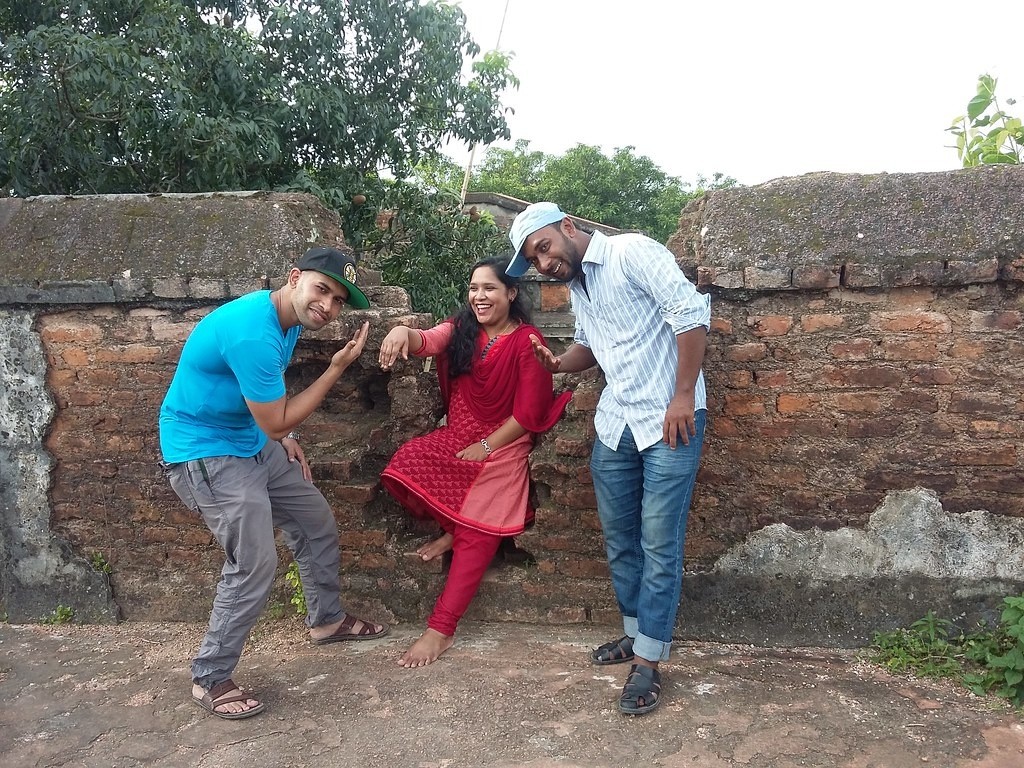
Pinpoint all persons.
[157,247,391,718]
[503,201,713,716]
[378,255,573,668]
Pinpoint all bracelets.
[284,431,300,441]
[480,439,493,456]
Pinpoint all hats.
[292,246,370,309]
[504,202,568,278]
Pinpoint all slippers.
[191,678,265,719]
[310,612,389,644]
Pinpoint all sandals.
[617,662,662,714]
[590,635,637,667]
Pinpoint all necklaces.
[489,318,515,340]
